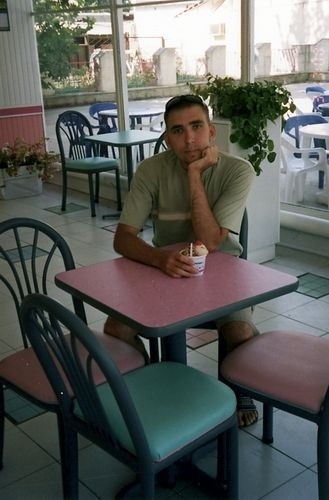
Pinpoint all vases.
[1,167,43,200]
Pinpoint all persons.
[104,94,260,429]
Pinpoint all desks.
[53,242,299,366]
[297,123,329,203]
[99,109,163,162]
[85,130,161,192]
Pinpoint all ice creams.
[182,240,208,256]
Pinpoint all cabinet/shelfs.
[209,116,281,265]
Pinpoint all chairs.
[0,86,329,500]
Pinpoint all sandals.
[236,395,258,427]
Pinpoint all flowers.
[1,136,62,184]
